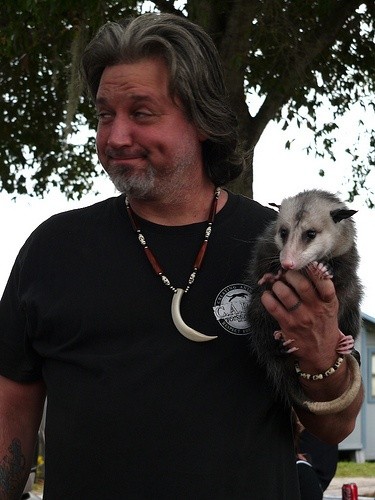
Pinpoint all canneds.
[342,483,358,500]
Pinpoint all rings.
[286,301,302,311]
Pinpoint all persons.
[0,14,364,500]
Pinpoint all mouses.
[240,191,363,409]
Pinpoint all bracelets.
[293,345,346,382]
[289,355,361,416]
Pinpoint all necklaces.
[122,185,224,342]
